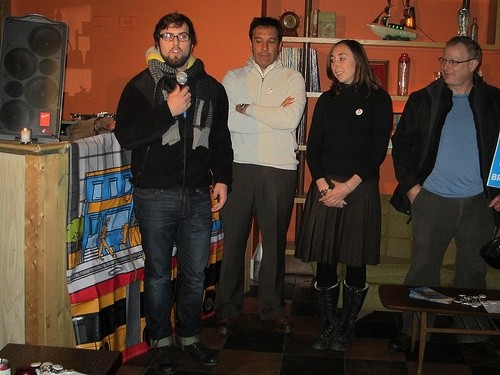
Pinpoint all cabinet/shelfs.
[260,0,500,254]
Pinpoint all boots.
[313,280,340,350]
[330,280,369,351]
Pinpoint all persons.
[388,38,500,352]
[114,11,235,375]
[294,41,394,352]
[212,16,307,336]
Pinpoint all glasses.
[437,57,479,66]
[159,32,192,42]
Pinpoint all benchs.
[312,193,500,319]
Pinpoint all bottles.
[470,18,478,43]
[397,53,410,96]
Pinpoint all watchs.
[320,190,328,196]
[240,104,246,113]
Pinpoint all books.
[277,47,320,92]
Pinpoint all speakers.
[0,14,69,142]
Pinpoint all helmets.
[480,237,500,270]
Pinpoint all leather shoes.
[151,344,175,375]
[267,312,295,333]
[216,316,234,335]
[179,342,217,366]
[389,333,427,353]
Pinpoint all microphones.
[176,71,187,119]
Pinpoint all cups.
[21,128,31,141]
[433,71,442,81]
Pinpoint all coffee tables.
[378,284,500,375]
[0,343,124,375]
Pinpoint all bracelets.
[344,183,352,192]
